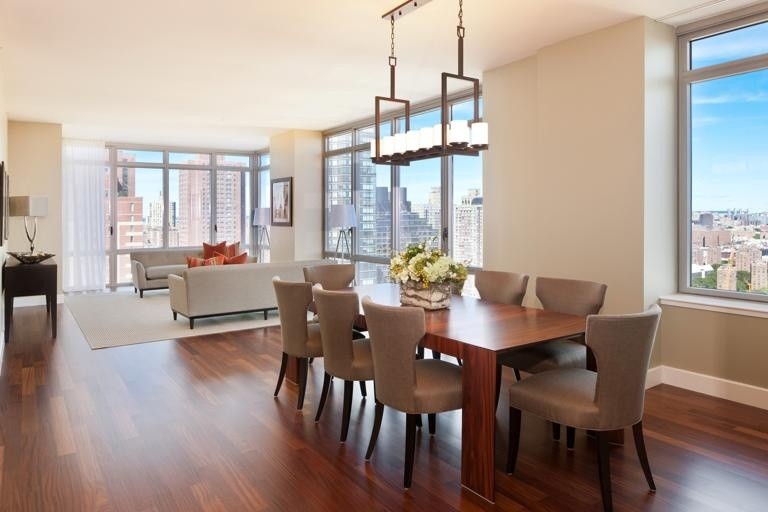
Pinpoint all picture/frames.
[271,176,292,226]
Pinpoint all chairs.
[537,276,607,438]
[313,282,375,442]
[360,294,463,490]
[506,303,662,511]
[474,270,529,380]
[272,275,365,410]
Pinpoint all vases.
[399,282,451,310]
[447,277,466,295]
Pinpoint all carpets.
[65,286,314,349]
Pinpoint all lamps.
[369,0,489,166]
[7,196,55,263]
[253,208,271,262]
[330,204,358,285]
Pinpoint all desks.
[310,284,586,510]
[4,255,56,342]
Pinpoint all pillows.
[186,241,247,268]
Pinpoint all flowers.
[449,262,470,276]
[387,235,452,282]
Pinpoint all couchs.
[168,258,338,329]
[130,248,258,298]
